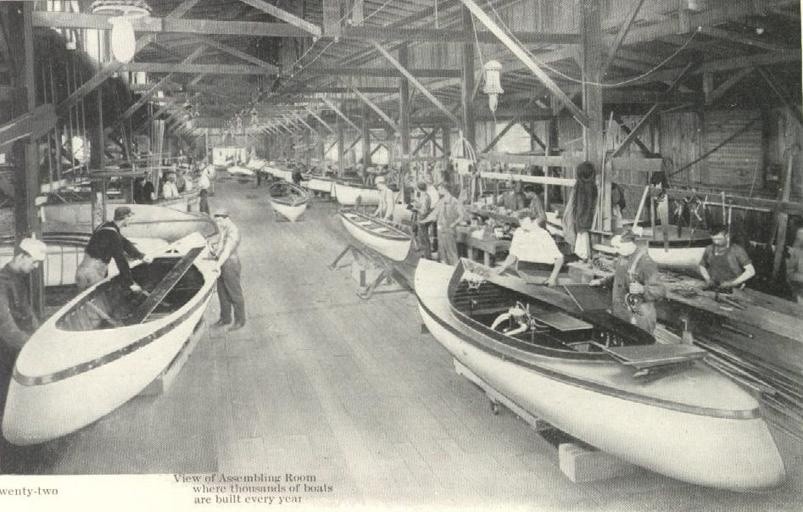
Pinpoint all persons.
[134,158,216,216]
[0,238,48,405]
[76,206,153,295]
[589,227,666,335]
[208,209,246,330]
[698,223,755,289]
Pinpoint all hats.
[115,206,135,217]
[19,237,47,261]
[215,207,229,216]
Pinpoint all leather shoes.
[215,318,244,330]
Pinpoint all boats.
[263,176,312,220]
[531,209,715,269]
[0,233,226,445]
[407,255,788,503]
[329,202,416,264]
[32,203,221,244]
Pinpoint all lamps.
[480,59,506,113]
[88,0,154,64]
